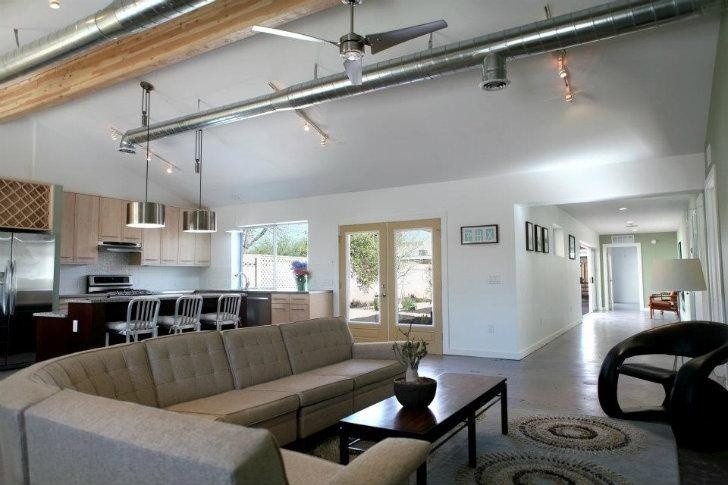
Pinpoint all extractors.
[100,240,143,253]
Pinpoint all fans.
[252,0,448,86]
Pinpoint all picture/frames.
[525,221,576,259]
[460,224,498,244]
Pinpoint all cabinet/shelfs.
[129,205,179,266]
[272,294,332,324]
[60,191,99,265]
[179,208,211,266]
[98,196,141,247]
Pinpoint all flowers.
[290,260,311,278]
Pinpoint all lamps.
[183,98,216,233]
[649,258,708,370]
[543,4,573,102]
[266,81,329,147]
[109,127,182,174]
[126,81,166,228]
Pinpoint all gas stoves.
[96,289,154,296]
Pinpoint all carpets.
[312,404,682,485]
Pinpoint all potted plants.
[391,340,437,410]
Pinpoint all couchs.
[0,315,430,485]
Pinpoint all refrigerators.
[0,232,57,371]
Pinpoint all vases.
[296,276,306,291]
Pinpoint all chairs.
[650,291,681,319]
[105,297,160,347]
[200,294,242,331]
[597,321,728,451]
[152,295,203,334]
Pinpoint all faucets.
[235,272,250,290]
